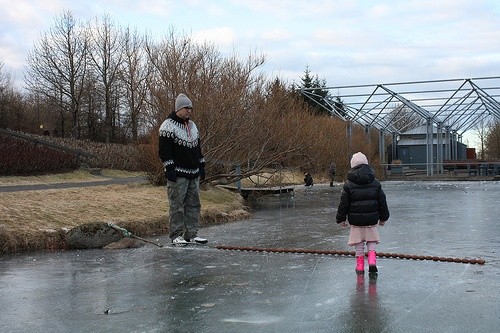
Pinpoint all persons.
[43,127,50,136]
[336,152,390,273]
[53,128,58,137]
[158,93,208,247]
[304,172,313,186]
[329,162,337,187]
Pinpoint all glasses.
[184,107,194,111]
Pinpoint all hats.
[351,152,369,168]
[175,93,193,112]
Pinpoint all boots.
[355,256,366,271]
[368,251,378,272]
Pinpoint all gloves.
[199,167,205,181]
[165,170,178,181]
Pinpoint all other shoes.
[184,236,208,244]
[172,236,189,246]
